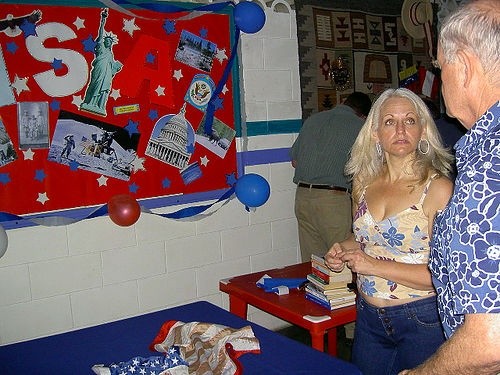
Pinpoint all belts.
[298,183,351,192]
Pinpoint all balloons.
[235,174,270,208]
[108,195,140,227]
[0,224,8,258]
[234,1,265,34]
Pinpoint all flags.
[399,63,441,100]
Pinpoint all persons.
[397,0,500,375]
[22,109,45,138]
[324,87,453,375]
[291,91,372,263]
[79,8,124,117]
[61,134,75,159]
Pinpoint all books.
[304,253,357,310]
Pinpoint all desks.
[0,301,362,375]
[219,257,357,357]
